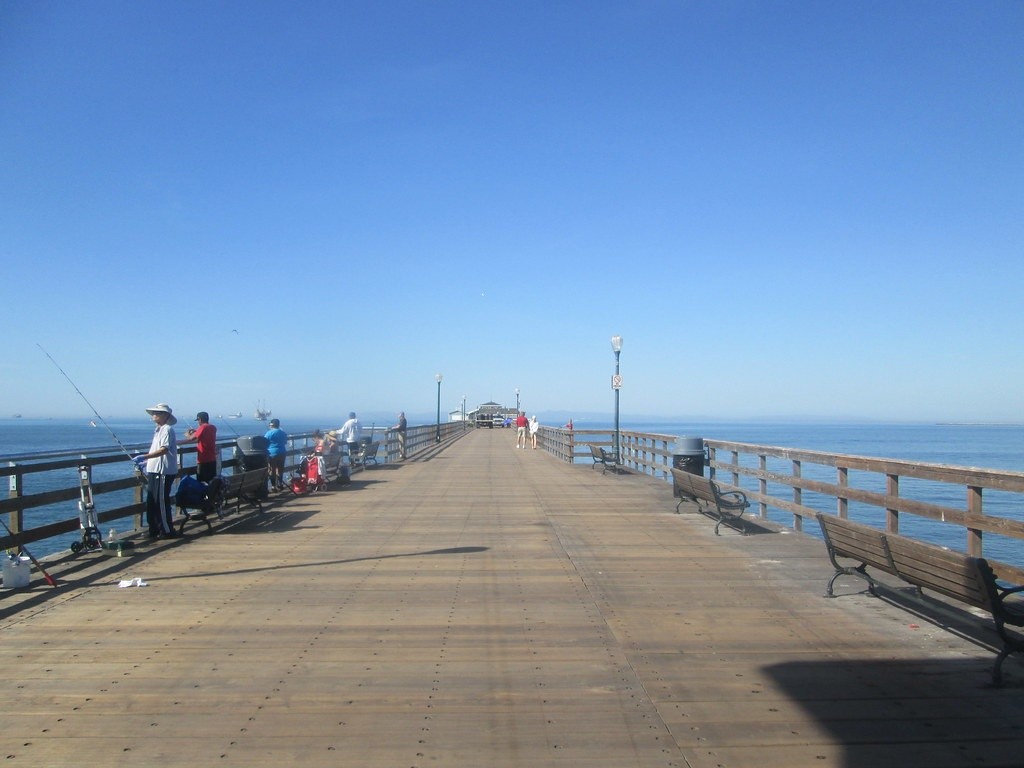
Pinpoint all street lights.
[515,387,520,432]
[436,372,443,443]
[460,393,466,431]
[610,333,623,466]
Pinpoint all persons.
[263,418,287,492]
[333,411,361,469]
[185,411,217,481]
[132,403,178,538]
[528,416,538,449]
[515,412,528,448]
[297,431,329,479]
[327,430,339,471]
[392,411,407,459]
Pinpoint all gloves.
[133,462,145,471]
[128,455,145,469]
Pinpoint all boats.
[230,413,243,418]
[255,398,271,421]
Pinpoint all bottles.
[108,528,117,541]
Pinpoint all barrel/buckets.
[2,556,30,587]
[340,466,351,479]
[291,478,306,493]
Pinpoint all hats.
[194,411,209,421]
[144,403,177,426]
[269,418,280,426]
[325,431,338,441]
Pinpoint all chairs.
[176,478,220,533]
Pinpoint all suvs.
[475,414,506,428]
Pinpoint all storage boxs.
[101,539,135,556]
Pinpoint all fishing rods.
[0,518,58,588]
[37,343,149,485]
[179,402,305,498]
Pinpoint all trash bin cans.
[670,436,707,499]
[233,435,271,500]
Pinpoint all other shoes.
[158,533,173,539]
[277,484,283,490]
[141,531,160,537]
[523,445,526,448]
[516,445,520,449]
[271,485,277,492]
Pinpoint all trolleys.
[297,446,327,492]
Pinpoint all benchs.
[348,441,380,471]
[587,443,619,475]
[219,467,270,514]
[815,511,1024,675]
[296,450,349,489]
[670,467,751,537]
